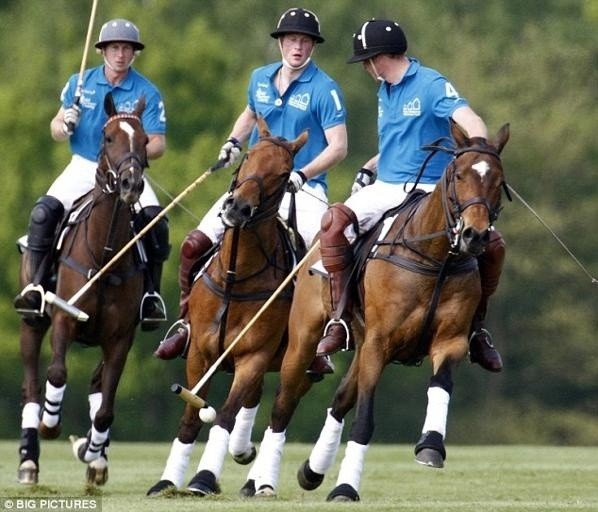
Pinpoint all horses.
[18,91,154,484]
[147,110,328,497]
[253,116,509,504]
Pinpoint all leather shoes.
[13,290,44,327]
[473,320,504,372]
[141,302,163,333]
[155,331,189,359]
[316,325,355,357]
[307,359,335,373]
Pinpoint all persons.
[14,20,166,330]
[151,9,349,376]
[315,19,506,373]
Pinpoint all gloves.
[351,168,373,194]
[286,170,303,193]
[62,107,80,137]
[218,140,242,168]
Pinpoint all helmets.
[95,20,144,51]
[271,8,324,43]
[347,20,407,63]
